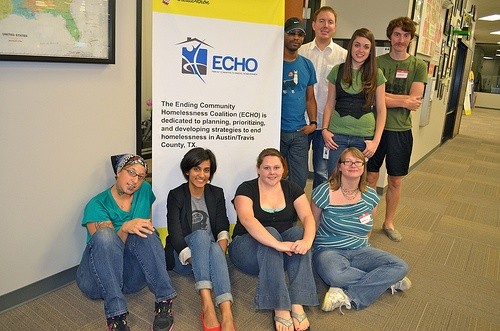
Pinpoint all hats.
[285,17,306,35]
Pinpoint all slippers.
[272,308,294,331]
[290,311,310,331]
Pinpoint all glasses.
[122,167,145,180]
[339,161,364,167]
[286,32,305,37]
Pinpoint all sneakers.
[321,287,351,315]
[152,299,174,331]
[107,313,131,331]
[388,276,412,294]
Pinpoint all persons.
[164,147,236,331]
[310,148,412,315]
[76,153,178,331]
[228,149,320,331]
[321,28,387,183]
[279,16,317,189]
[365,17,428,242]
[297,6,349,190]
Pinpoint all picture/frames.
[0,0,115,64]
[433,0,478,100]
[411,0,425,25]
[407,33,419,56]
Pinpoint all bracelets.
[309,121,318,128]
[321,128,328,132]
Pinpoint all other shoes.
[201,310,221,331]
[383,226,402,242]
